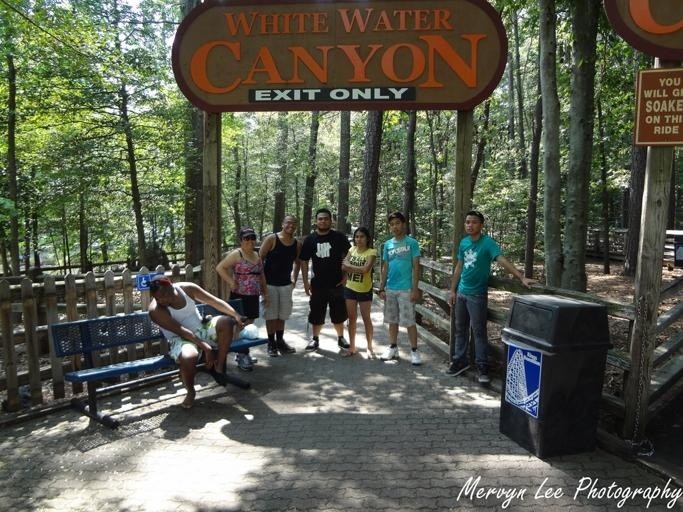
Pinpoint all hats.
[240,227,257,238]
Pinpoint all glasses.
[243,237,256,241]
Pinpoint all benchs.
[49,297,269,427]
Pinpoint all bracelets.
[263,293,270,298]
[379,287,385,294]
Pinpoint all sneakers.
[411,350,421,364]
[445,359,470,376]
[210,366,227,387]
[238,353,254,372]
[235,354,258,363]
[268,344,278,357]
[341,349,357,358]
[476,364,491,383]
[305,336,319,352]
[380,345,399,360]
[367,349,376,361]
[276,340,295,353]
[338,336,350,349]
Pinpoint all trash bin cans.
[499,295,614,458]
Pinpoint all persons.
[149,274,244,409]
[445,211,538,383]
[258,215,302,357]
[299,209,352,354]
[341,227,378,360]
[379,212,423,365]
[216,226,270,371]
